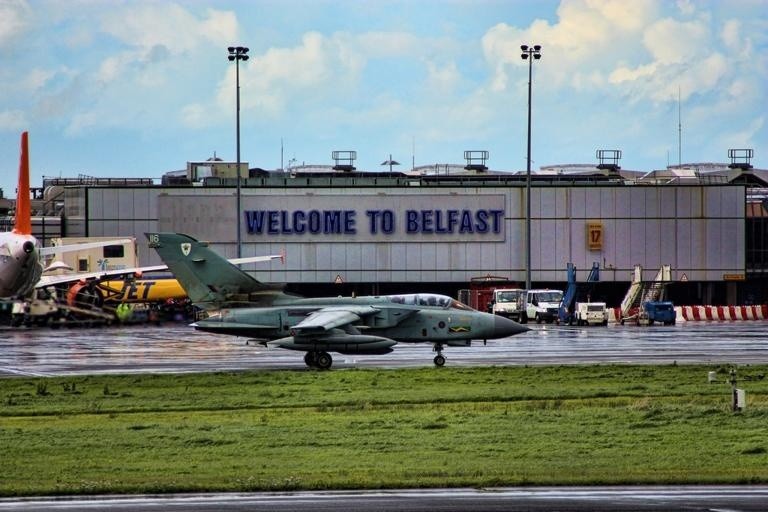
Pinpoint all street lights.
[518,44,542,291]
[226,45,249,266]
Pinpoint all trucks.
[458,284,565,322]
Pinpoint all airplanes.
[0,128,286,333]
[145,230,534,371]
